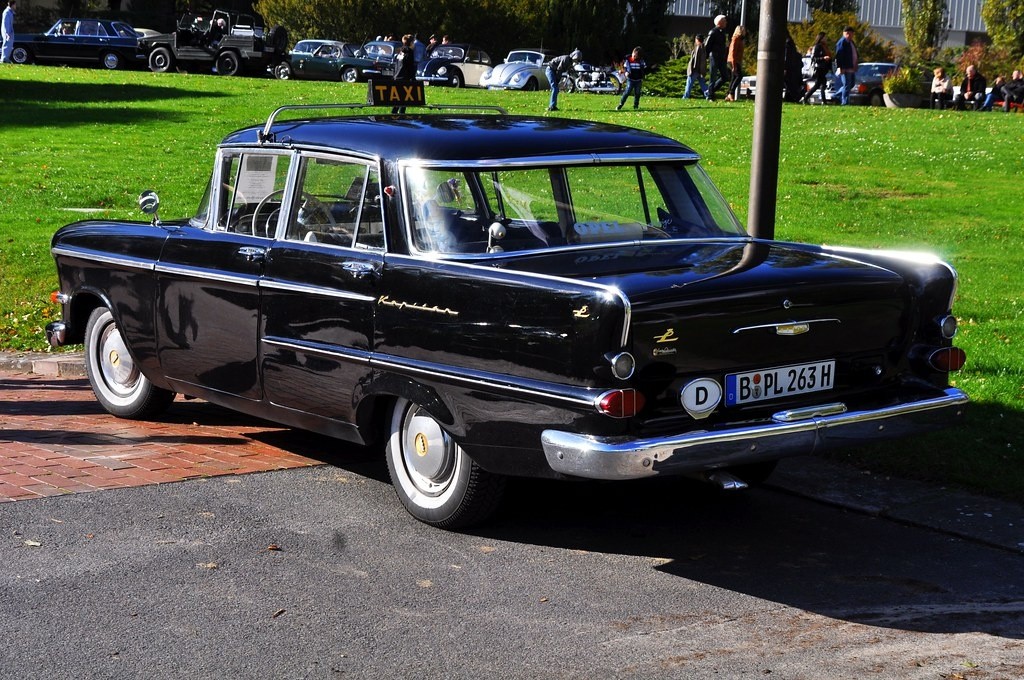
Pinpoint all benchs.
[457,212,645,257]
[574,71,621,94]
[923,84,1024,114]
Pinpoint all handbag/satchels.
[808,58,818,79]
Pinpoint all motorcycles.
[559,59,622,95]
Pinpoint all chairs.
[304,229,385,248]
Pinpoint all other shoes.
[616,104,624,110]
[549,106,560,112]
[724,97,735,102]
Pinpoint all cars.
[354,40,403,61]
[739,55,834,101]
[45,114,971,527]
[413,43,493,88]
[0,18,146,70]
[119,28,162,61]
[803,63,934,108]
[270,39,383,83]
[477,47,558,91]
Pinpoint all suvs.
[137,8,287,76]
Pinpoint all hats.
[714,15,726,25]
[844,26,854,32]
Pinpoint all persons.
[371,34,453,115]
[979,70,1024,112]
[929,67,954,111]
[682,34,710,100]
[0,0,16,64]
[545,51,582,110]
[797,32,832,104]
[61,26,72,34]
[956,64,986,112]
[831,26,858,106]
[724,25,747,103]
[190,16,227,73]
[703,15,730,101]
[616,46,647,110]
[785,29,802,103]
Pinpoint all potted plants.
[882,65,927,109]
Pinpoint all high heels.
[799,98,810,105]
[821,101,828,106]
